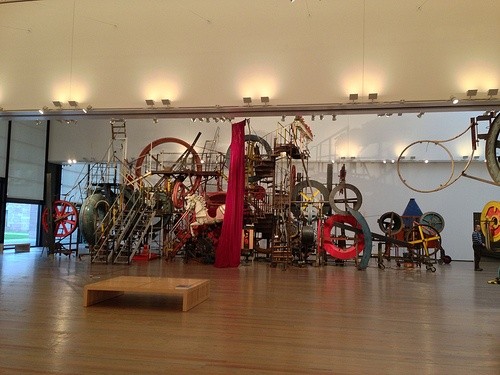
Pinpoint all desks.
[83,275,208,312]
[0,242,31,254]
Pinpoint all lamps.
[483,89,499,115]
[39,106,48,115]
[462,155,480,161]
[281,115,287,122]
[33,119,96,164]
[162,99,171,108]
[243,97,252,105]
[191,117,235,123]
[451,97,459,104]
[311,115,355,159]
[369,93,378,102]
[82,105,93,114]
[377,112,425,159]
[145,100,155,107]
[260,97,270,105]
[68,101,79,109]
[153,119,159,124]
[349,94,358,103]
[466,89,478,102]
[52,101,63,109]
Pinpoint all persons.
[472,225,485,271]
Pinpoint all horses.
[185,192,225,237]
